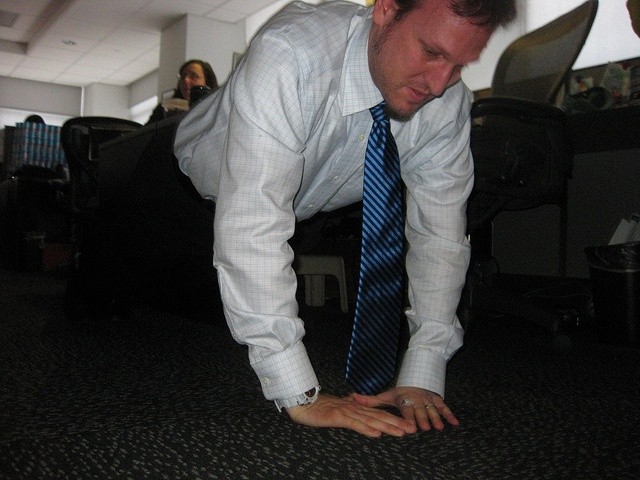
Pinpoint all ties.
[345,101,405,396]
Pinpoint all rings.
[425,403,433,409]
[400,399,414,409]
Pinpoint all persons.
[144,59,222,129]
[48,0,515,449]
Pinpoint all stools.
[294,255,348,315]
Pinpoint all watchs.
[272,384,321,413]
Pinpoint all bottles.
[189,85,211,108]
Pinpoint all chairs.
[18,117,144,275]
[459,0,598,351]
